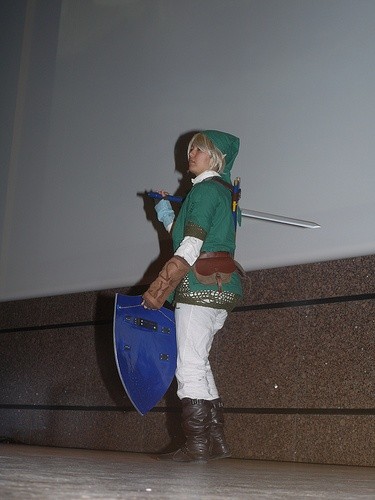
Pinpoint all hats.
[200,130,239,184]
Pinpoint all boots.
[156,397,213,461]
[206,398,231,460]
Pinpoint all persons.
[138,131,243,464]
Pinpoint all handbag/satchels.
[195,252,237,293]
